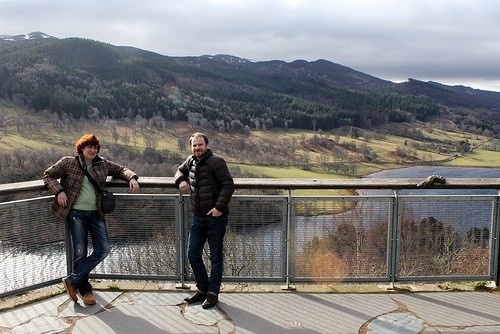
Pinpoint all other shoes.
[62,278,78,303]
[83,292,96,305]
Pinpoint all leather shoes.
[184,290,207,303]
[202,293,218,308]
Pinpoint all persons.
[43,134,139,304]
[175,133,235,308]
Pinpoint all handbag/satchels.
[101,190,115,214]
